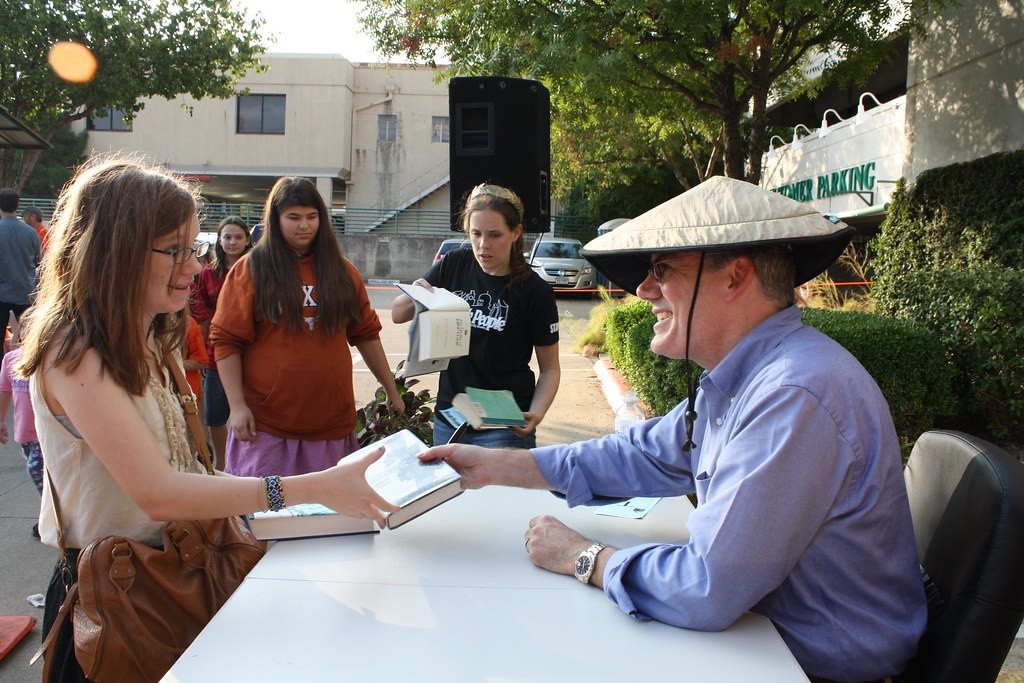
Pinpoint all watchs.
[574,543,605,583]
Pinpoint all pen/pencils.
[448,421,468,443]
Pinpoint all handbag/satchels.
[69,516,266,683]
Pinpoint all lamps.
[819,109,845,139]
[792,124,812,150]
[856,92,883,126]
[769,135,786,158]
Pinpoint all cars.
[522,236,596,298]
[432,239,471,274]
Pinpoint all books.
[247,503,380,541]
[338,429,464,530]
[394,284,471,378]
[452,387,525,429]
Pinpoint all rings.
[525,539,530,551]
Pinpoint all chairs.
[903,430,1024,683]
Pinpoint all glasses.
[153,239,211,264]
[647,252,728,279]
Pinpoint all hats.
[577,175,857,296]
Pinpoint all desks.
[159,484,811,682]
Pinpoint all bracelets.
[265,475,286,511]
[258,476,263,510]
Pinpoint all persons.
[392,177,561,448]
[20,164,399,683]
[22,207,48,246]
[207,175,407,476]
[0,188,41,371]
[0,308,43,539]
[183,217,264,470]
[417,175,927,683]
